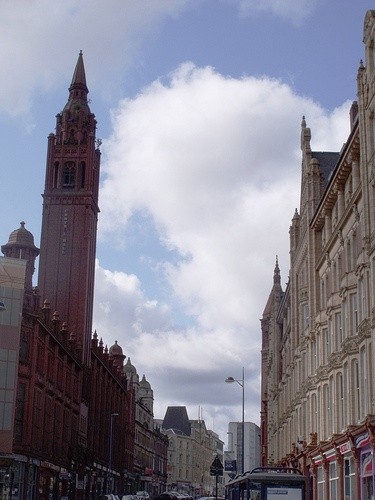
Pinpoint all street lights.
[108,413,119,491]
[225,366,245,474]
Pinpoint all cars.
[102,490,193,500]
